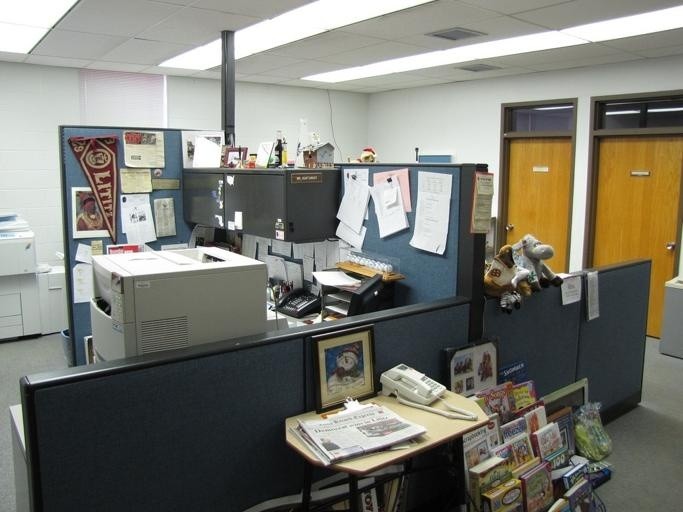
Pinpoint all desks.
[284,387,489,511]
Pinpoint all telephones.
[380,364,447,406]
[275,287,321,318]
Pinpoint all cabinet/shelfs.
[184,169,224,229]
[225,170,339,240]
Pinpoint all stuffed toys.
[353,148,376,163]
[484,233,563,315]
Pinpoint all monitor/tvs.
[347,274,384,317]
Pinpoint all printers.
[91,245,268,363]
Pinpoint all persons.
[76,194,103,230]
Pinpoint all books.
[462,380,600,512]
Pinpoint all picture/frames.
[440,337,498,392]
[223,147,247,168]
[179,129,225,169]
[305,323,379,412]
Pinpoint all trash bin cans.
[61,329,75,367]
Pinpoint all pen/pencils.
[280,280,293,294]
